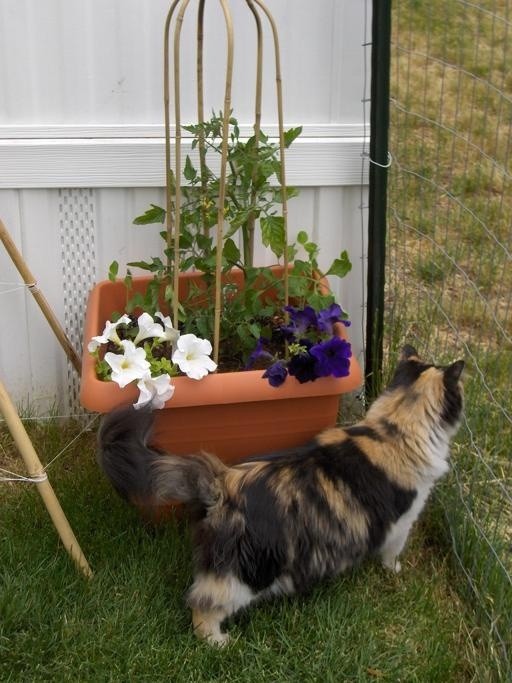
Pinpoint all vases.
[72,264,365,480]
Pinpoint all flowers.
[84,107,363,422]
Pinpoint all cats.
[98,343,466,651]
